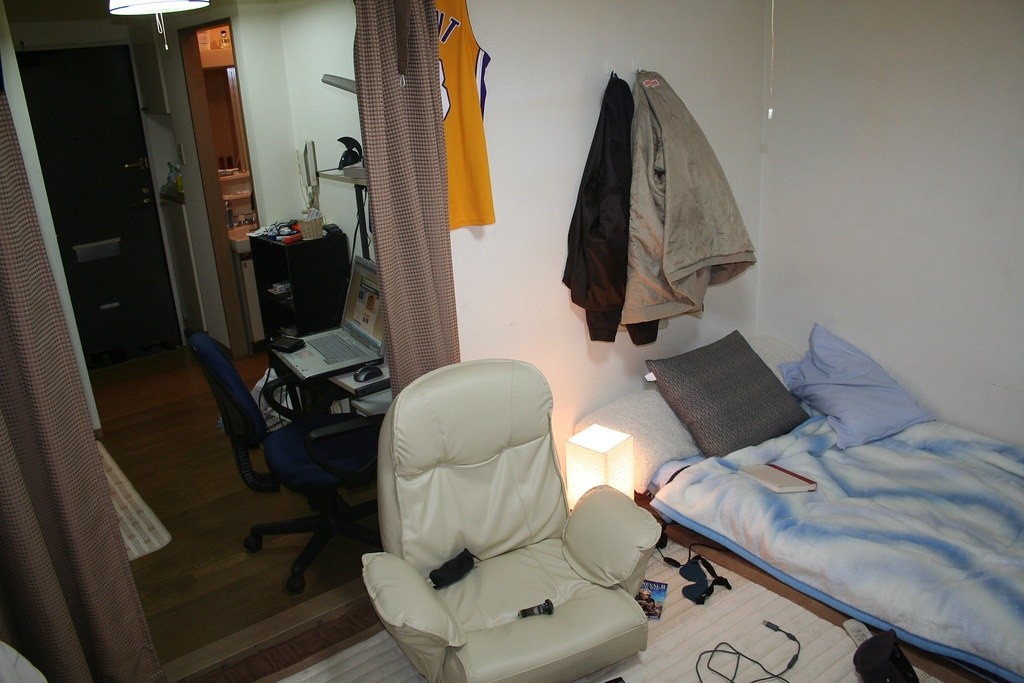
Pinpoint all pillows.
[573,334,805,494]
[780,320,936,450]
[646,331,811,458]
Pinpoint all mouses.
[354,366,383,382]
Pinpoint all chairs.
[360,359,662,683]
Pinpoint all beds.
[647,413,1024,683]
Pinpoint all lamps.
[108,0,211,50]
[563,422,635,511]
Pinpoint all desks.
[267,325,393,415]
[186,329,382,595]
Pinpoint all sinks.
[227,223,257,253]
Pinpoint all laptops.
[272,255,385,382]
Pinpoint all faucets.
[233,221,248,227]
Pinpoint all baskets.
[299,216,324,240]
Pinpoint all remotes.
[843,619,873,647]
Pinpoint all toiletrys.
[218,167,238,174]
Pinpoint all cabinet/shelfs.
[247,230,351,376]
